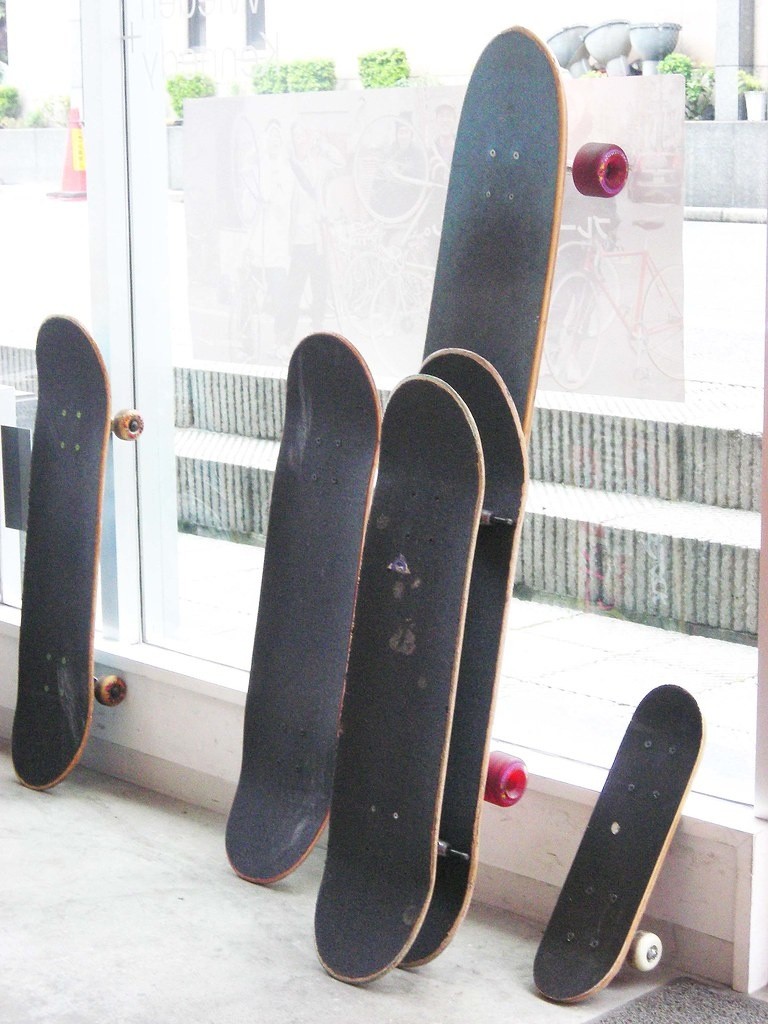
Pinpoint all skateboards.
[9,319,143,792]
[534,685,706,1009]
[227,29,627,982]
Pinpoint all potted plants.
[357,48,408,88]
[32,97,76,182]
[0,86,32,183]
[739,73,766,122]
[164,73,214,192]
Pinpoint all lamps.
[582,19,632,77]
[546,23,592,77]
[629,20,683,75]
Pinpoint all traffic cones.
[43,107,85,200]
